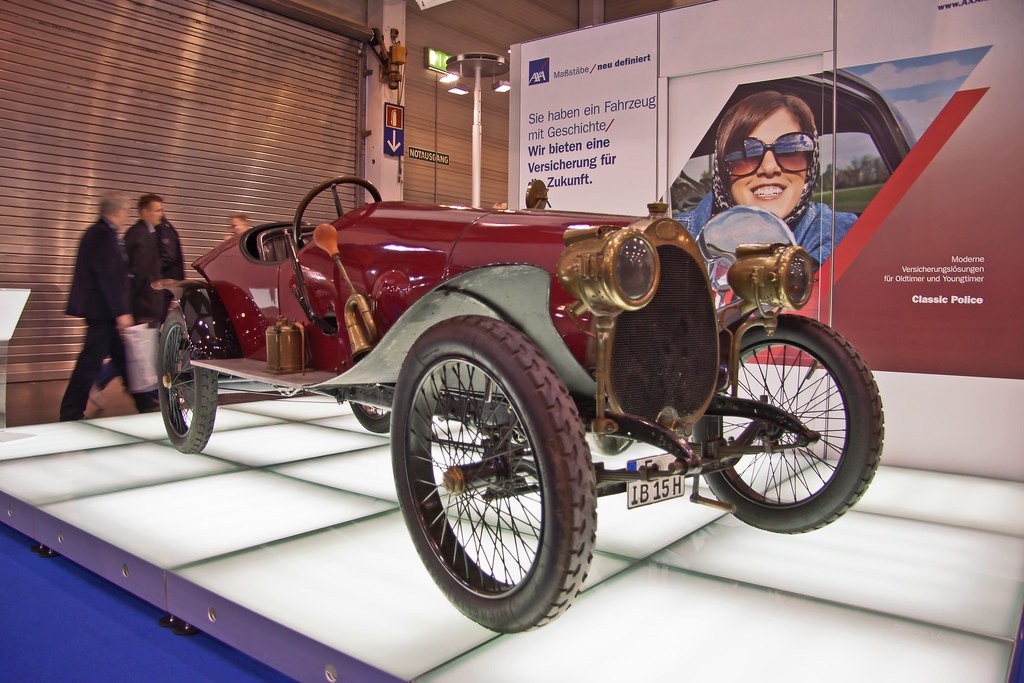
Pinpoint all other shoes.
[139,403,160,413]
[89,384,107,410]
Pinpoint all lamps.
[447,83,472,96]
[492,80,511,93]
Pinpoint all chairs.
[256,225,318,264]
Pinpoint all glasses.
[724,132,816,177]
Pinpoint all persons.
[59,193,184,422]
[673,91,858,309]
[224,212,250,240]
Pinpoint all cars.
[158,177,888,634]
[671,69,919,332]
